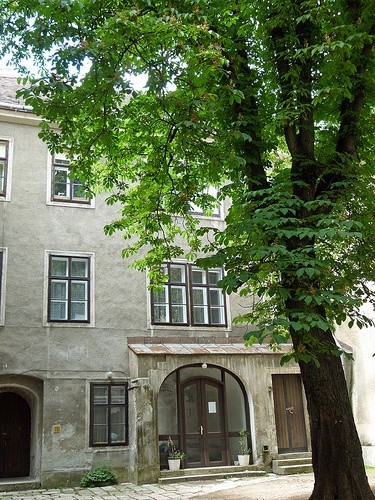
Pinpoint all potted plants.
[237,428,252,466]
[234,460,239,466]
[79,467,120,487]
[167,450,187,471]
